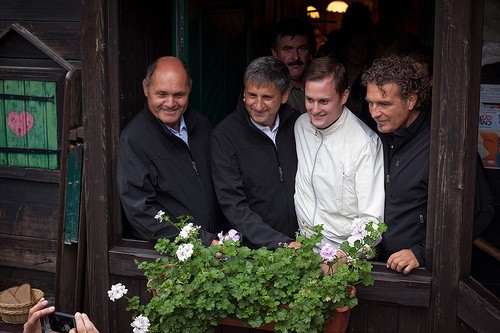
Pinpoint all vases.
[218,285,357,333]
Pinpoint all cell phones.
[48,312,76,333]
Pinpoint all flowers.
[106,209,389,333]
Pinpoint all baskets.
[0,284,44,324]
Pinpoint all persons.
[208,16,434,276]
[23,296,99,333]
[111,54,222,248]
